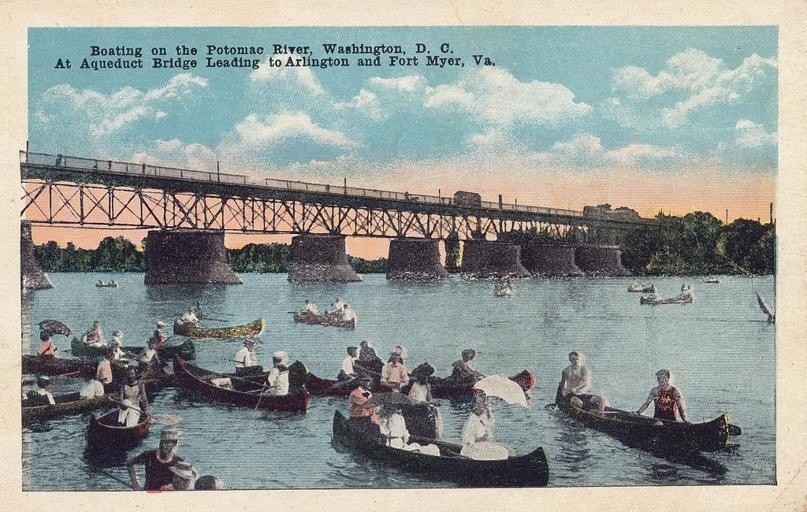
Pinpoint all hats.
[161,424,179,443]
[168,460,197,482]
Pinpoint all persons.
[22,271,723,491]
